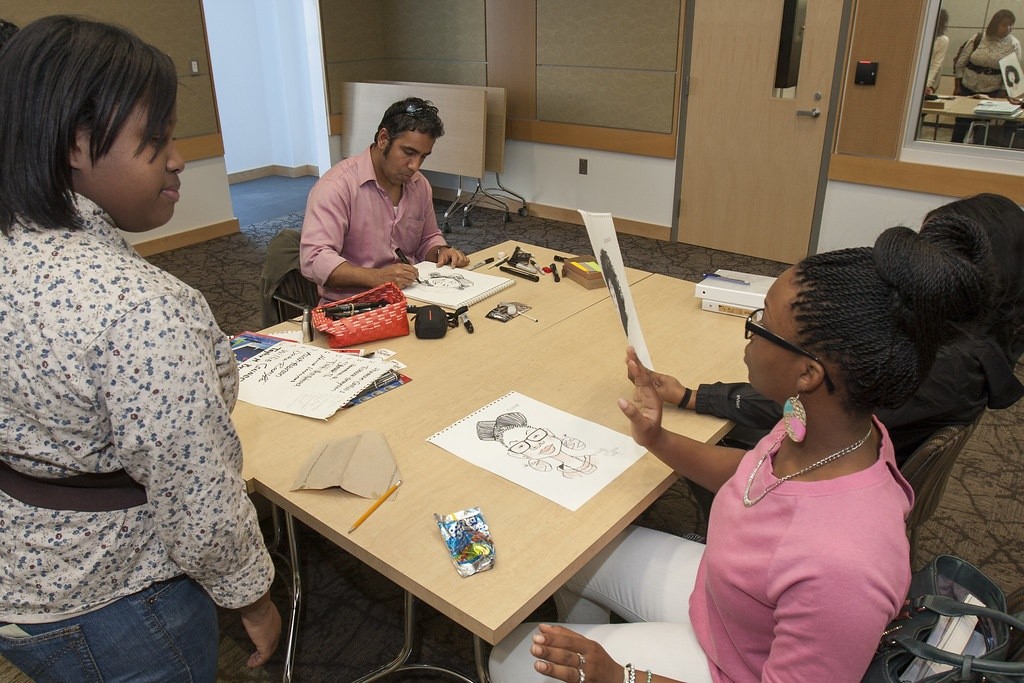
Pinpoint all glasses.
[388,103,434,121]
[745,308,836,395]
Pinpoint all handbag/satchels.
[953,32,983,73]
[861,553,1024,683]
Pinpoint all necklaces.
[743,422,873,508]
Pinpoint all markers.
[550,263,560,282]
[322,300,395,319]
[530,259,545,275]
[395,247,425,286]
[508,259,536,274]
[460,311,475,333]
[467,255,495,271]
[500,266,539,283]
[341,369,400,406]
[554,255,568,262]
[361,352,375,360]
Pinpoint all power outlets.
[189,56,200,75]
[578,159,587,175]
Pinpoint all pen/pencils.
[517,311,539,322]
[348,480,405,534]
[701,271,750,285]
[489,255,509,270]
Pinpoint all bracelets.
[434,245,461,261]
[623,663,635,683]
[678,387,692,409]
[647,670,652,683]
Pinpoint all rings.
[577,669,585,683]
[576,653,586,668]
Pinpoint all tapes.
[300,306,314,343]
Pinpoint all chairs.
[899,409,982,572]
[260,228,321,327]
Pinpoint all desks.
[922,96,1024,149]
[342,79,526,232]
[178,242,756,683]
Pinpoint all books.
[400,261,517,311]
[974,100,1023,119]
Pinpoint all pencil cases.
[310,281,410,348]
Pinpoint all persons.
[488,215,996,683]
[950,9,1022,143]
[0,14,281,683]
[1008,96,1024,109]
[299,97,470,307]
[923,8,949,117]
[625,192,1024,522]
